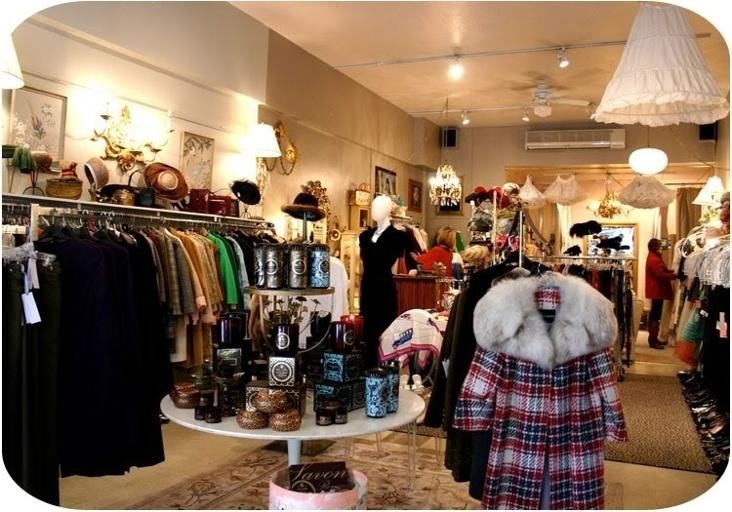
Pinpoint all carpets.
[126,436,621,509]
[389,371,719,476]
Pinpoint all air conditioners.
[523,128,628,152]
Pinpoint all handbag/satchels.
[190,188,239,217]
[101,184,155,207]
[46,178,82,199]
[470,240,492,253]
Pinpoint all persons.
[644,238,678,350]
[435,225,465,279]
[358,195,407,333]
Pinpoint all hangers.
[499,236,517,264]
[539,258,629,271]
[4,201,264,237]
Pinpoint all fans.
[503,82,592,110]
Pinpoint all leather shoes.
[677,368,729,474]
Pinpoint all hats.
[144,162,188,203]
[281,193,326,221]
[84,157,109,190]
[228,180,261,204]
[32,152,60,175]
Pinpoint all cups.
[216,309,363,354]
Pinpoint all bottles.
[252,241,332,291]
[366,365,400,416]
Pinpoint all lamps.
[534,103,553,118]
[556,48,571,68]
[82,88,174,173]
[428,97,463,206]
[462,111,470,125]
[244,118,281,162]
[688,166,726,207]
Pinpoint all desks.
[158,376,428,468]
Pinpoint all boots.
[648,322,666,349]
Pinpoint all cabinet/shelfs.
[351,205,410,230]
[392,275,450,316]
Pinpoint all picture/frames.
[407,178,423,213]
[179,131,215,191]
[434,176,464,217]
[7,84,69,162]
[375,165,397,198]
[582,222,639,294]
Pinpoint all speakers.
[699,120,717,142]
[443,127,458,150]
